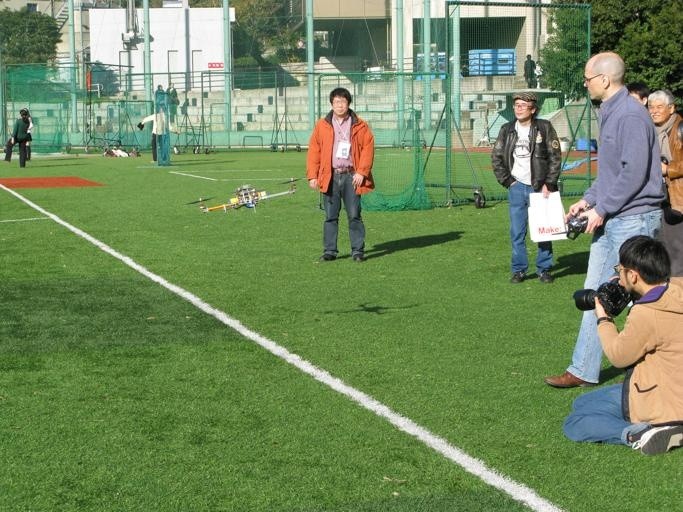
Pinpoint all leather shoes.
[353,254,363,261]
[318,254,336,260]
[543,372,595,387]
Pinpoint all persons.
[648,89,683,214]
[4,109,32,167]
[545,51,665,388]
[306,87,375,262]
[626,81,650,107]
[563,235,683,456]
[23,108,33,160]
[490,92,561,284]
[524,54,537,88]
[142,107,180,163]
[154,85,166,112]
[166,83,180,124]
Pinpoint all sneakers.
[510,271,526,283]
[640,426,683,456]
[539,270,552,282]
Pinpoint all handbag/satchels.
[528,191,568,243]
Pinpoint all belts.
[332,167,353,174]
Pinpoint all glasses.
[512,104,533,109]
[614,265,633,273]
[584,73,604,83]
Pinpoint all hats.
[513,92,538,102]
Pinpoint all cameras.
[567,216,588,241]
[573,278,633,317]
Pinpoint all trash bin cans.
[558,136,570,153]
